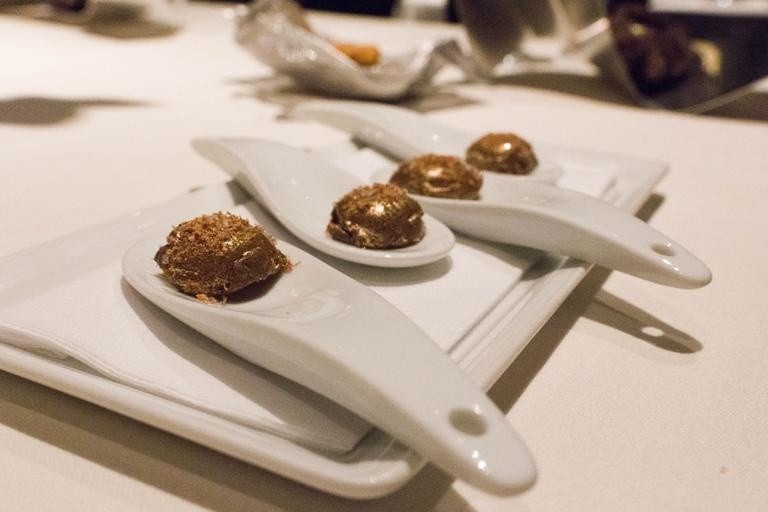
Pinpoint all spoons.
[292,98,567,187]
[388,153,713,290]
[190,131,456,270]
[121,220,537,496]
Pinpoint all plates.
[0,73,671,502]
